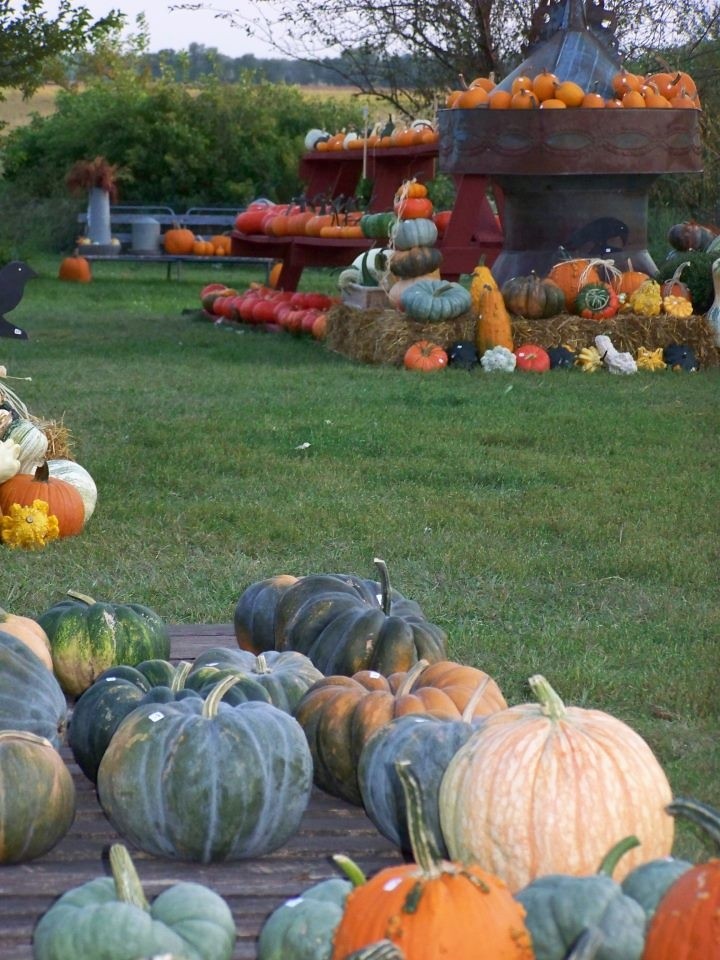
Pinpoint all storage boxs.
[342,280,389,312]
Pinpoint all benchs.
[72,204,287,286]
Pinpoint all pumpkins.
[0,367,98,547]
[58,66,719,375]
[0,554,720,959]
